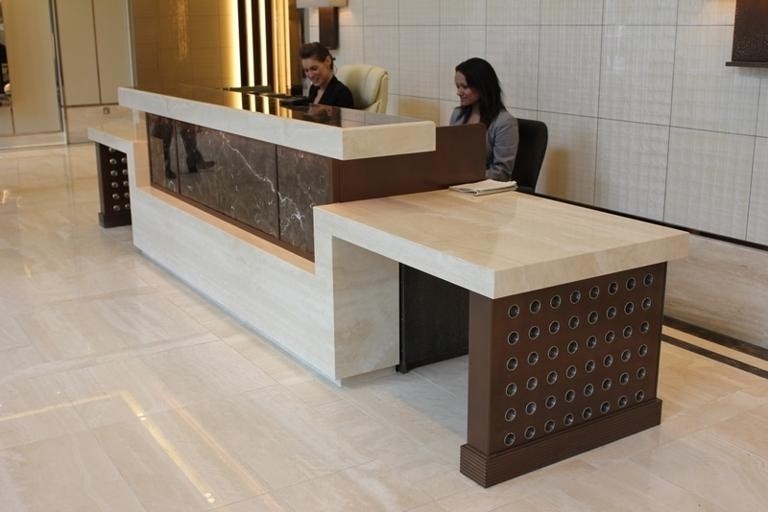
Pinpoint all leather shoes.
[165,169,177,180]
[187,156,216,173]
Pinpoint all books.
[446,178,518,198]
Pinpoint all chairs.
[512,119,549,194]
[335,63,388,112]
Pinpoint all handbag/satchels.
[152,118,165,139]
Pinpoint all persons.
[303,101,335,124]
[151,115,216,180]
[297,41,358,112]
[446,56,521,182]
